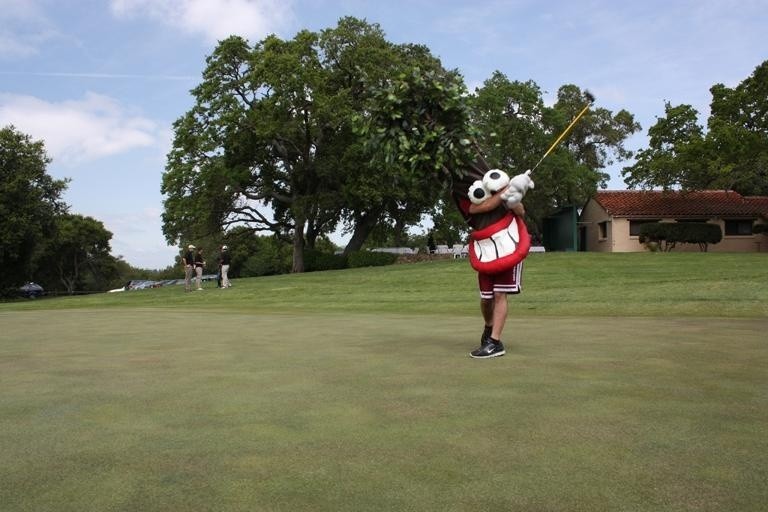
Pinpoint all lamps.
[371,245,545,260]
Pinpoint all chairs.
[183,290,196,295]
[220,284,233,289]
[196,287,204,291]
[469,325,506,358]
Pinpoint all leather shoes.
[501,168,536,209]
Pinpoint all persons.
[183,244,196,293]
[218,245,232,289]
[193,247,206,291]
[427,235,436,263]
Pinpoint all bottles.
[221,245,229,250]
[188,244,196,250]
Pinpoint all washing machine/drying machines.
[501,92,595,211]
